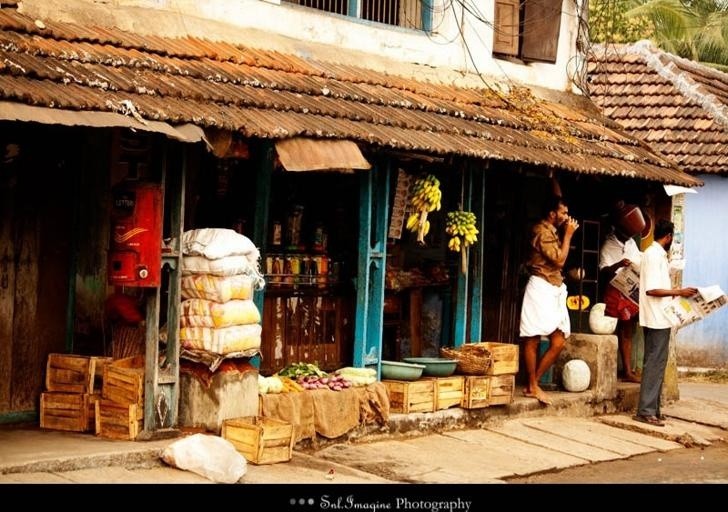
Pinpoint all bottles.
[270,204,327,250]
[266,254,338,286]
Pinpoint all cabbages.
[258,374,283,394]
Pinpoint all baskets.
[439,344,492,376]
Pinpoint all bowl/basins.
[381,360,425,380]
[404,357,458,377]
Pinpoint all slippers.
[631,415,663,426]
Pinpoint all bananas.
[406,173,441,237]
[445,212,478,252]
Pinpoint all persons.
[634,218,696,426]
[598,217,642,384]
[517,197,580,406]
